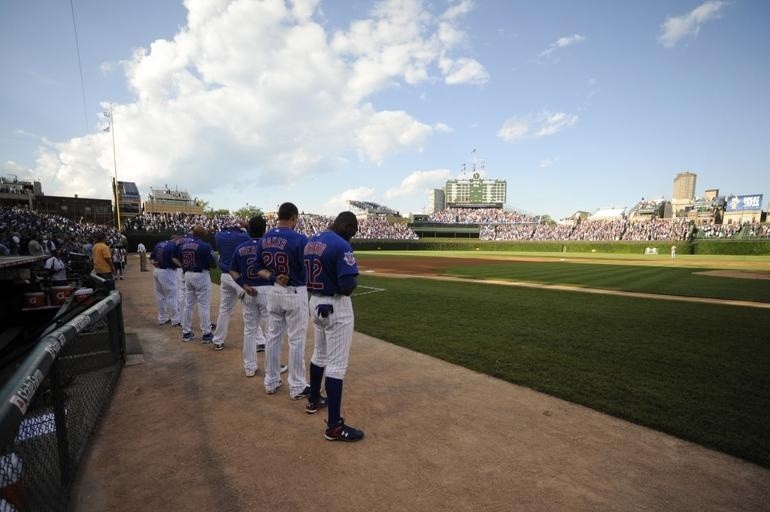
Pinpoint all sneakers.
[213,341,225,351]
[181,330,196,342]
[324,421,364,443]
[305,396,329,414]
[244,367,257,377]
[158,318,184,330]
[255,343,265,351]
[264,364,288,394]
[201,332,215,344]
[291,383,323,400]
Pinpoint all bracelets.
[235,277,247,288]
[268,273,278,283]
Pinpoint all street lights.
[96,100,121,233]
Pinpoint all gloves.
[314,302,336,320]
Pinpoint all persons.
[301,210,366,444]
[152,219,220,344]
[670,244,678,260]
[0,199,130,297]
[252,201,313,401]
[211,217,268,353]
[136,239,150,272]
[226,216,289,378]
[127,184,422,244]
